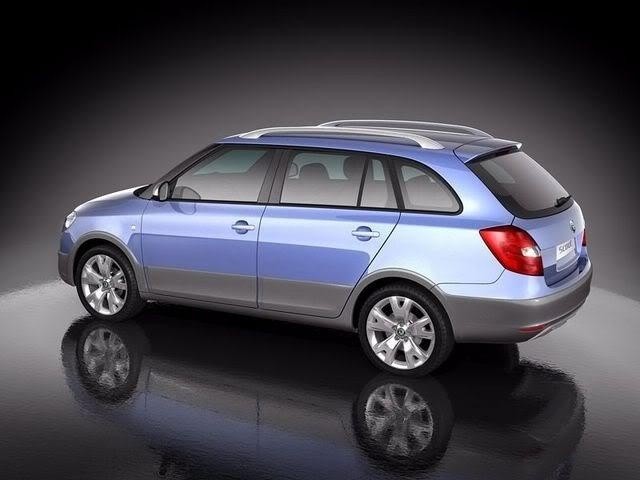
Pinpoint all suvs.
[60,314,588,480]
[50,117,591,382]
